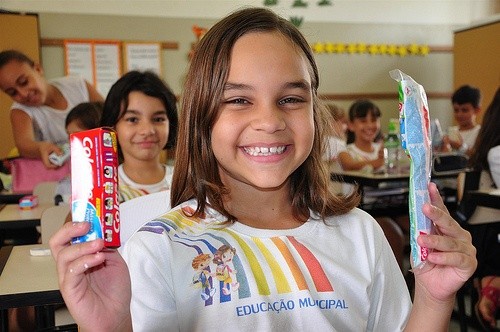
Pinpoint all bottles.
[384,122,401,176]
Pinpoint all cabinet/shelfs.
[455,18,499,131]
[0,12,42,154]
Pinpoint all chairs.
[32,176,66,200]
[426,148,475,219]
[41,201,85,326]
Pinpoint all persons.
[320,103,354,198]
[447,85,481,149]
[55,101,105,205]
[338,100,410,275]
[458,86,500,332]
[48,7,478,332]
[0,50,105,169]
[100,70,178,205]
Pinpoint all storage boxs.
[69,128,122,252]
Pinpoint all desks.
[1,136,499,332]
